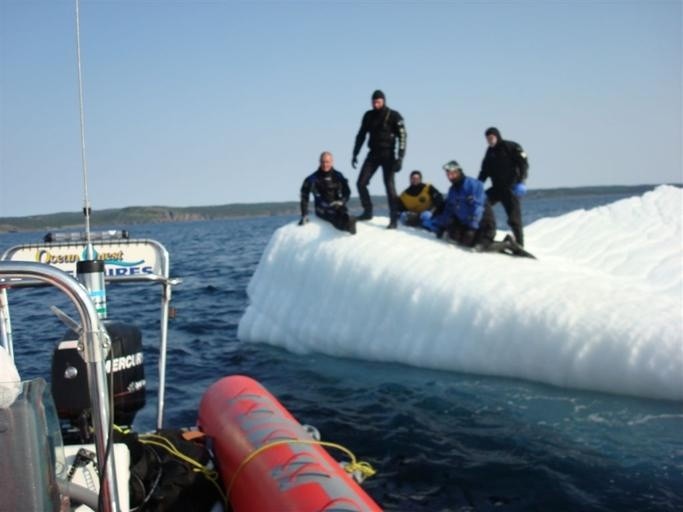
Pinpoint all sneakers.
[347,217,357,234]
[503,234,537,261]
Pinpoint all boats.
[0,221,382,512]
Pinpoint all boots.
[386,211,400,229]
[355,209,374,221]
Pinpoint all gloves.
[351,158,359,168]
[329,199,344,209]
[437,225,449,238]
[420,209,433,221]
[298,214,310,225]
[512,182,528,198]
[458,227,475,246]
[391,159,402,172]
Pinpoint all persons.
[430,161,537,260]
[298,152,357,234]
[395,171,443,233]
[352,89,407,229]
[477,126,528,247]
